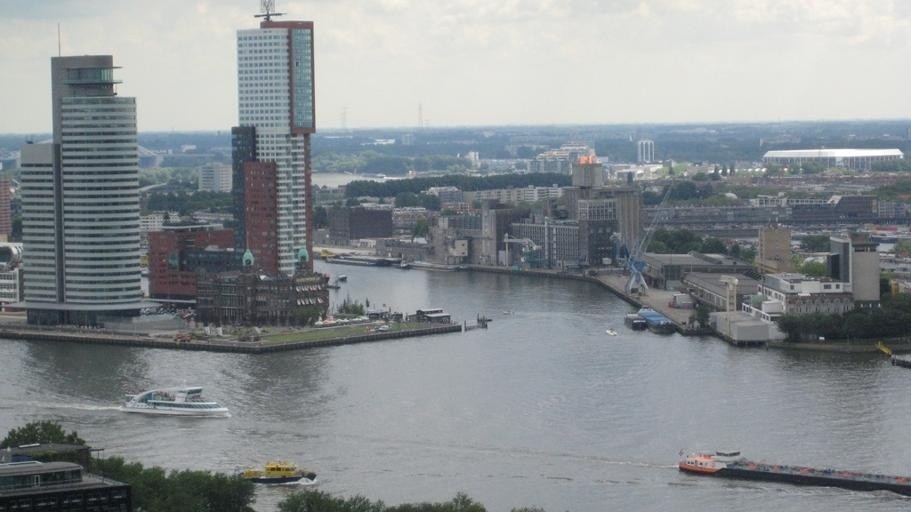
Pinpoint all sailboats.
[397,247,410,269]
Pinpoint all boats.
[624,311,647,330]
[640,304,677,334]
[328,282,341,290]
[676,448,911,496]
[242,460,319,484]
[605,328,620,336]
[115,384,234,420]
[336,273,349,281]
